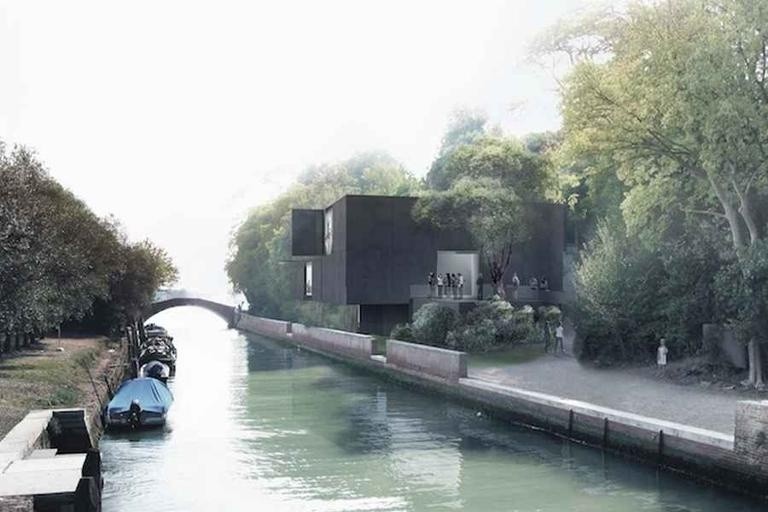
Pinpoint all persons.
[554,321,566,353]
[428,271,549,300]
[544,320,552,354]
[654,338,669,378]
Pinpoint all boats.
[106,324,177,427]
